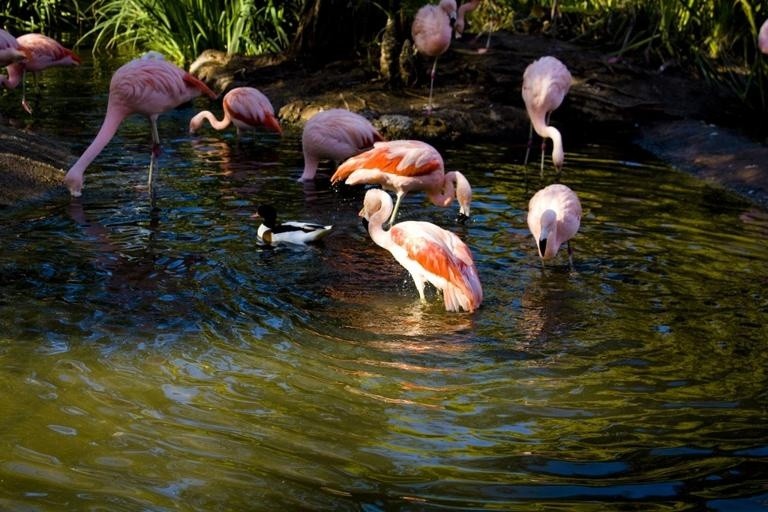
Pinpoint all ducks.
[250,204,334,247]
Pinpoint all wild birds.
[328,139,473,226]
[0,28,82,115]
[526,183,583,278]
[410,0,458,116]
[188,86,283,137]
[294,108,387,182]
[357,188,483,315]
[454,0,481,36]
[61,59,217,198]
[521,55,573,181]
